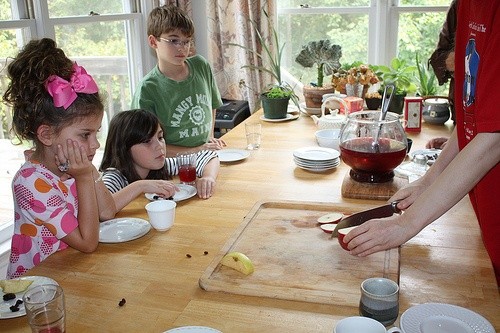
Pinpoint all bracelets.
[95,171,106,184]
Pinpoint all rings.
[56,160,70,173]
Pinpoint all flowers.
[331,65,377,90]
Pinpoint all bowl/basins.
[339,108,408,181]
[315,128,350,153]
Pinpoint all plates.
[145,183,197,202]
[214,147,250,162]
[98,217,151,244]
[162,325,222,333]
[0,275,59,320]
[400,302,497,333]
[291,147,341,172]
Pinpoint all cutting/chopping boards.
[197,197,403,308]
[341,166,414,198]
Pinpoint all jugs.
[419,94,453,125]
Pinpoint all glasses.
[159,37,195,47]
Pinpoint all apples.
[220,252,254,275]
[317,214,363,250]
[0,279,33,293]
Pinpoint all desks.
[0,105,500,333]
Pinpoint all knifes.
[330,199,410,239]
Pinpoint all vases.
[346,83,363,99]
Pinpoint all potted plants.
[217,2,306,120]
[294,32,344,108]
[339,64,379,97]
[378,57,416,113]
[416,53,447,97]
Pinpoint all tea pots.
[311,96,350,133]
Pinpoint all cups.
[176,151,197,185]
[333,316,402,333]
[145,199,176,231]
[245,121,262,150]
[359,277,400,327]
[23,284,65,333]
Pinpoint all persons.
[98,107,220,214]
[428,0,457,129]
[0,37,117,283]
[138,3,227,158]
[340,0,500,292]
